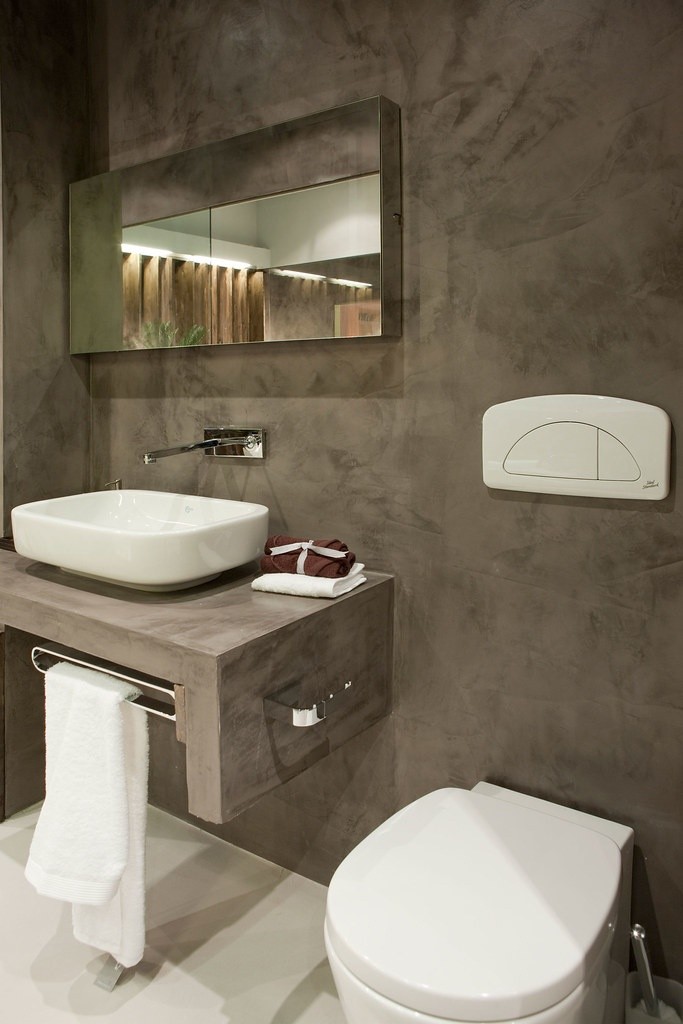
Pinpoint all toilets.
[324,781,634,1024]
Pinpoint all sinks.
[11,488,267,592]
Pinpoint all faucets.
[144,439,221,464]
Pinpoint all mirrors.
[65,95,404,354]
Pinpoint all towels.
[23,661,150,969]
[251,535,368,598]
